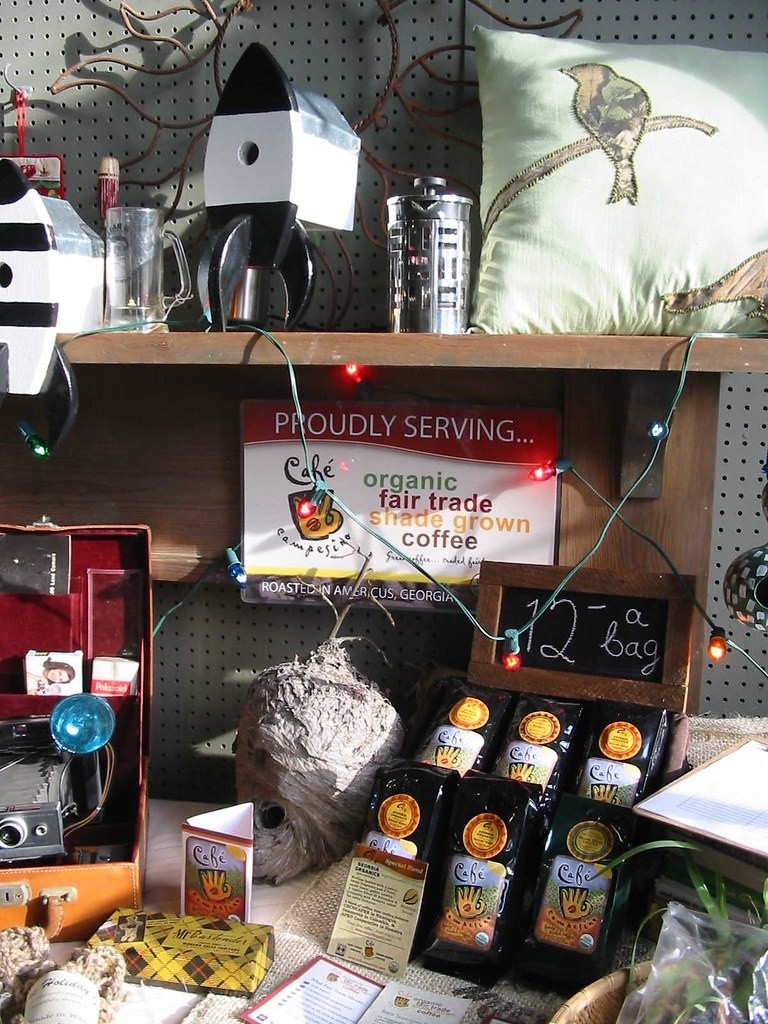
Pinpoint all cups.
[104,207,192,331]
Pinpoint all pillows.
[467,23,768,335]
